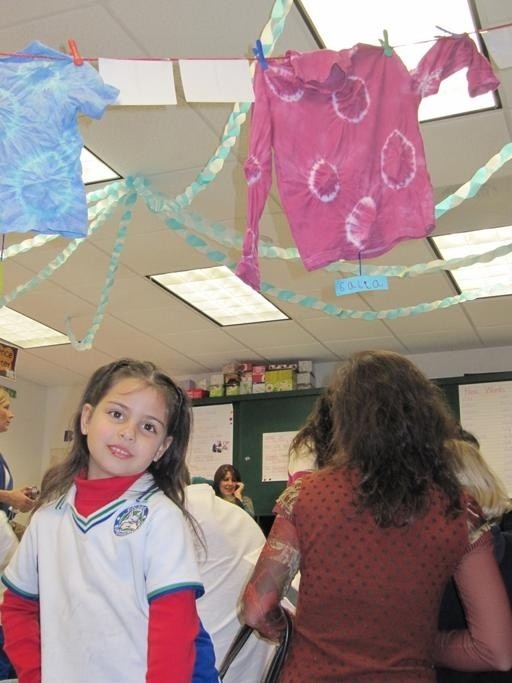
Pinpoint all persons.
[430,426,512,683]
[171,458,269,683]
[0,358,223,683]
[0,386,40,680]
[214,463,255,519]
[235,348,512,683]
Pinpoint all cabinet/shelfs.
[183,372,512,517]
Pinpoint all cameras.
[28,487,41,500]
